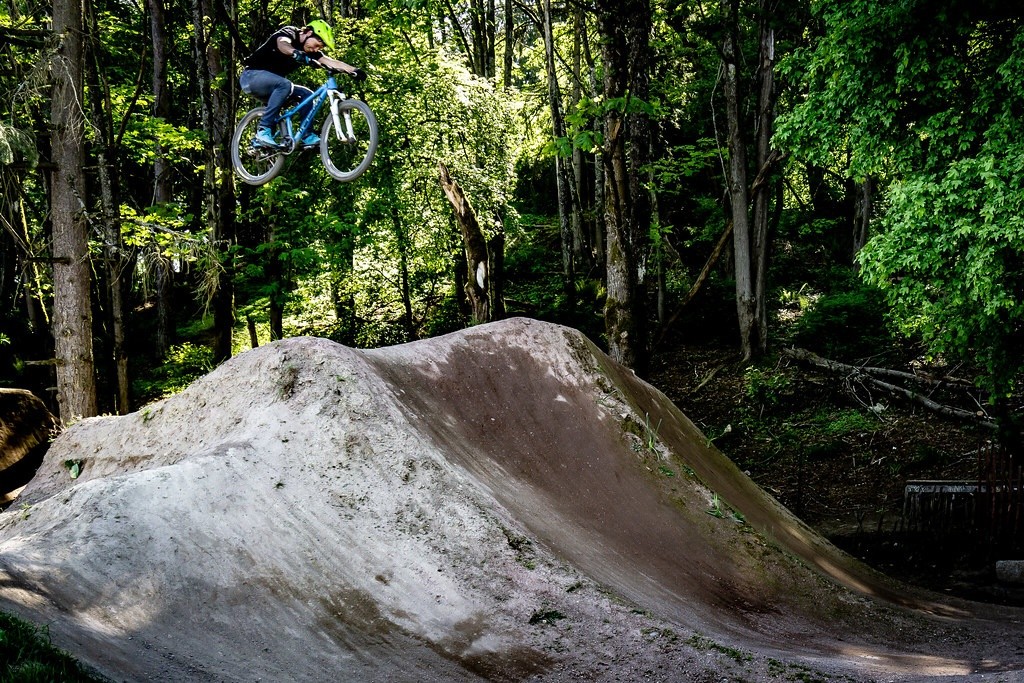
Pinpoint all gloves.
[292,50,309,66]
[349,68,367,80]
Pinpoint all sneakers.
[255,126,278,148]
[299,133,321,146]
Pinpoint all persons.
[239,20,367,149]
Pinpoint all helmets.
[306,19,335,51]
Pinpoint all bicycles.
[231,53,379,186]
